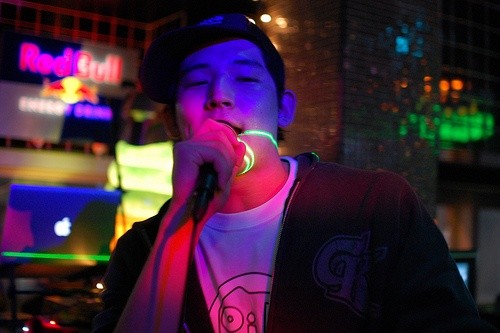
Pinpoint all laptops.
[0,184,122,260]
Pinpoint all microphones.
[190,120,237,223]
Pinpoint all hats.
[141,14,284,104]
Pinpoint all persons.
[88,13,486,333]
